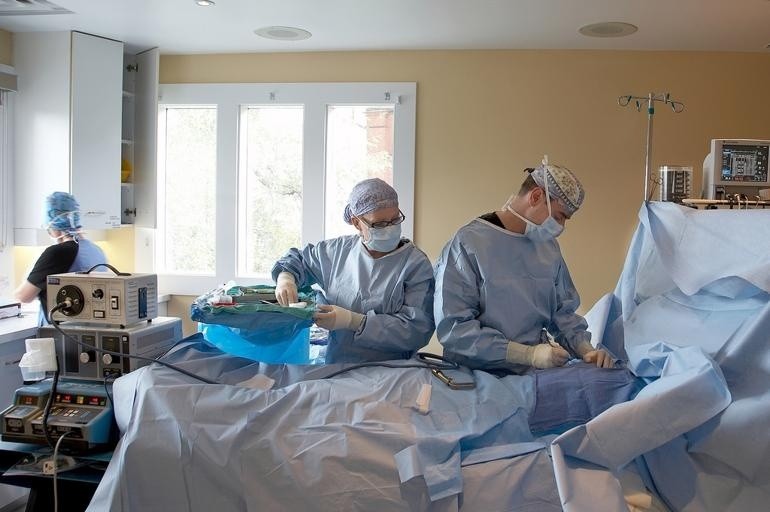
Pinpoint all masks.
[359,215,401,253]
[522,198,565,246]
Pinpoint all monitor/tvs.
[711,139,770,187]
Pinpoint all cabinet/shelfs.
[14,28,160,230]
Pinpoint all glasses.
[359,208,406,229]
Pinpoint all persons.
[433,163,616,370]
[15,192,108,337]
[528,342,724,512]
[272,178,436,362]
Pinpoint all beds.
[84,200,770,512]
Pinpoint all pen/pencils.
[543,327,572,361]
